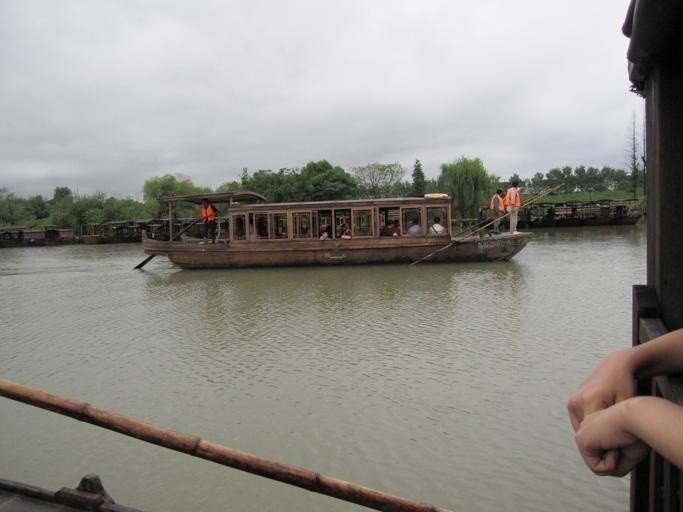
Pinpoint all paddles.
[133,215,208,268]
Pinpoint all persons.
[567,328,683,477]
[429,216,443,234]
[490,181,524,235]
[380,220,400,237]
[319,231,331,240]
[407,218,420,233]
[199,198,218,244]
[341,223,352,239]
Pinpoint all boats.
[479,198,642,229]
[1,220,140,247]
